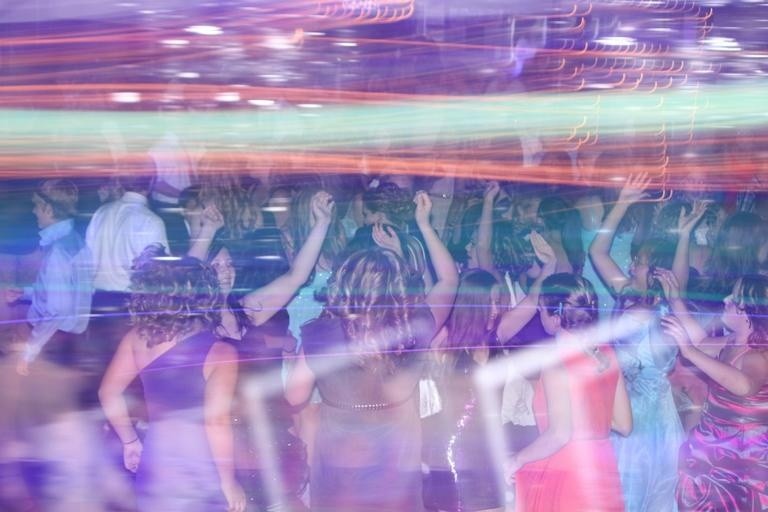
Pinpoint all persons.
[5,139,768,512]
